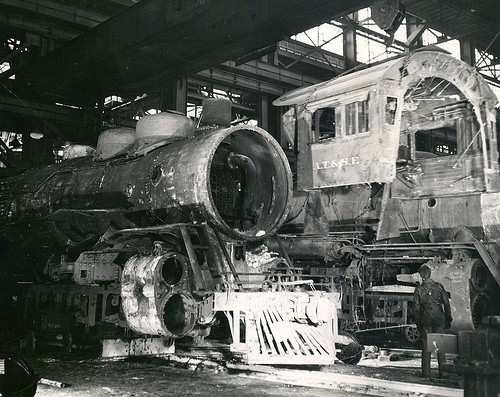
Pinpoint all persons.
[410,265,452,377]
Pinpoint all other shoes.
[440,372,450,378]
[420,373,428,377]
